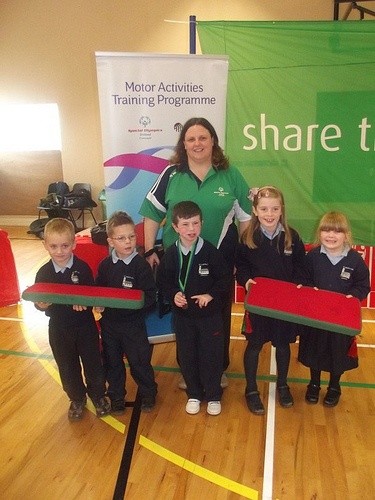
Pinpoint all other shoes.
[323,383,341,407]
[110,398,125,416]
[186,398,200,415]
[178,376,188,390]
[306,381,320,403]
[221,373,230,388]
[276,381,294,407]
[245,386,265,416]
[207,401,221,416]
[67,397,87,420]
[142,388,157,411]
[93,396,112,418]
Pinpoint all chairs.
[62,183,98,231]
[38,181,70,228]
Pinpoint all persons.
[33,217,111,419]
[298,213,370,407]
[138,117,253,389]
[235,187,305,414]
[157,201,231,416]
[94,212,156,412]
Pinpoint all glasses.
[111,233,136,241]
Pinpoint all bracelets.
[143,249,154,256]
